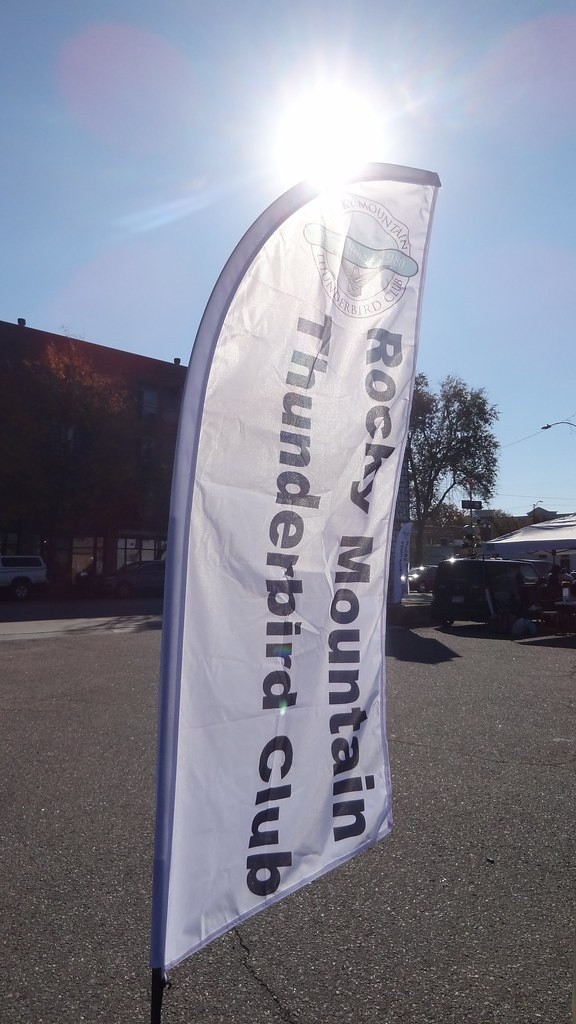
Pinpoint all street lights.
[533,500,543,523]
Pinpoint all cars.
[431,557,576,625]
[402,565,438,593]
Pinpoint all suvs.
[102,561,166,599]
[0,556,52,601]
